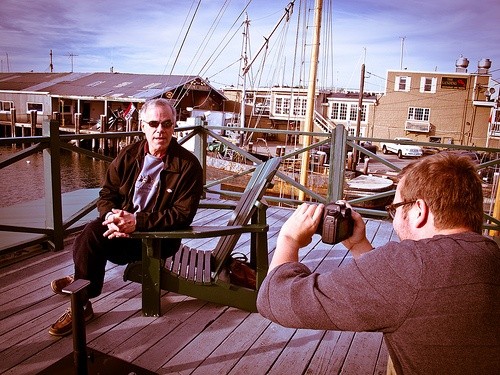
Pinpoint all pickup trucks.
[381,136,423,159]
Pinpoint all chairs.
[121,156,283,318]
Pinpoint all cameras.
[313,200,353,244]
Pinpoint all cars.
[294,139,377,162]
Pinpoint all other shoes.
[48,301,95,338]
[50,273,75,296]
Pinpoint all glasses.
[384,199,417,220]
[141,119,174,128]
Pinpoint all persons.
[48,98,203,336]
[257,152,499,375]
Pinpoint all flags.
[122,103,135,120]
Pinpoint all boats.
[345,175,395,209]
